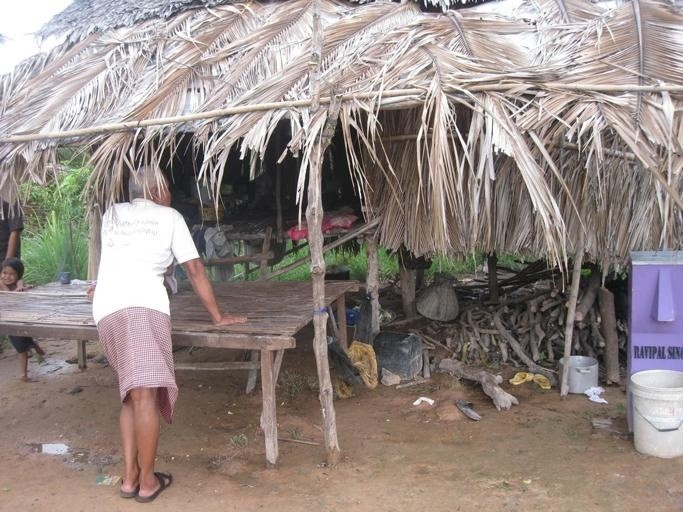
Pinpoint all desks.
[0,277,359,470]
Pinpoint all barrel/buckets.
[374,329,423,381]
[628,370,683,459]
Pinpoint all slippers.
[509,372,534,385]
[533,374,552,390]
[456,399,481,420]
[120,472,171,502]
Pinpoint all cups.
[59,272,71,283]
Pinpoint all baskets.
[416,272,458,322]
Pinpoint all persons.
[0,193,21,265]
[85,161,248,503]
[0,257,44,382]
